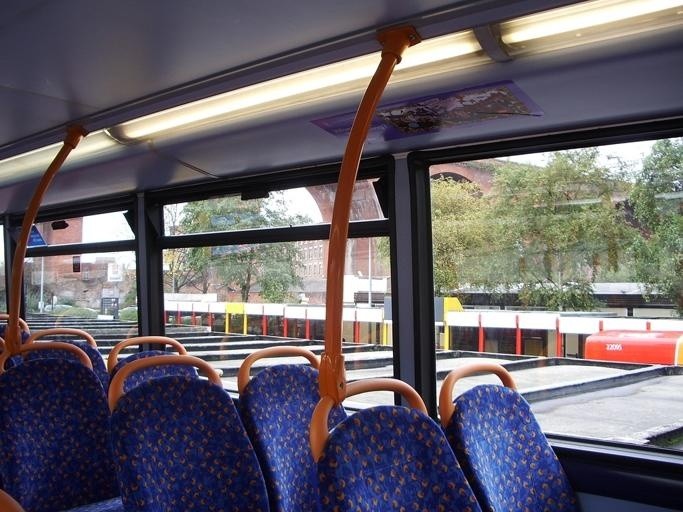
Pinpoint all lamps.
[107,15,492,187]
[490,0,683,58]
[0,127,131,187]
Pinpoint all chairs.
[1,342,123,512]
[436,360,579,512]
[108,336,197,392]
[23,328,106,371]
[106,354,269,512]
[308,381,481,511]
[238,346,347,512]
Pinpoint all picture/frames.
[309,79,545,147]
